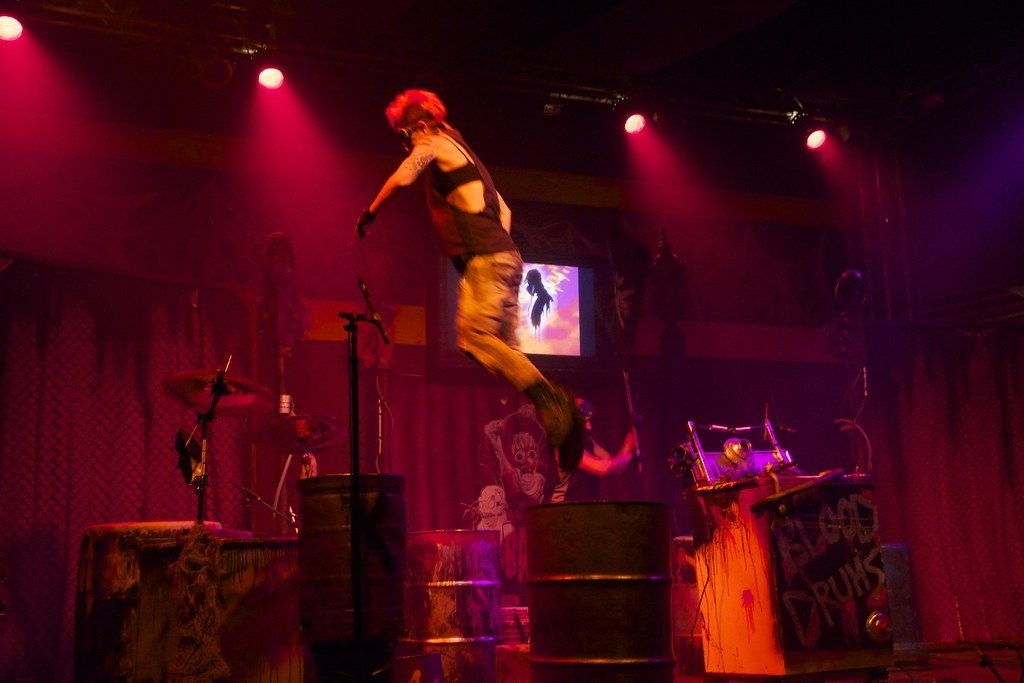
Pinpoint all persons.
[356,90,584,472]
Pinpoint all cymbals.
[162,369,276,414]
[253,415,347,456]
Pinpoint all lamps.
[611,95,645,134]
[786,110,827,151]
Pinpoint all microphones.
[764,403,769,440]
[273,336,290,415]
[862,367,869,396]
[357,278,390,345]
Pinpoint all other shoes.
[559,404,583,473]
[524,383,572,447]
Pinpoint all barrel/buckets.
[526,503,672,683]
[300,475,404,643]
[404,529,500,683]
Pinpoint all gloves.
[356,210,377,239]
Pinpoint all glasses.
[396,124,416,158]
